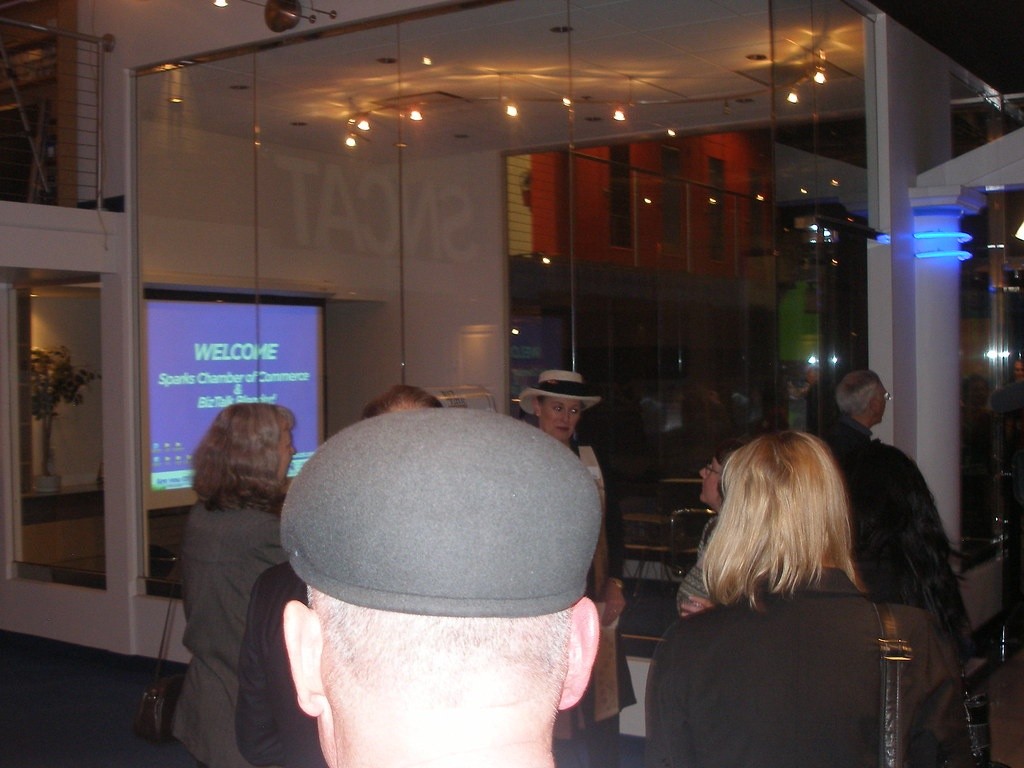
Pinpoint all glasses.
[877,393,893,401]
[704,463,722,479]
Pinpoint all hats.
[519,370,601,414]
[279,409,600,616]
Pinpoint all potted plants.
[19,344,102,493]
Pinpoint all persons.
[671,435,771,618]
[827,441,997,768]
[233,383,450,768]
[817,369,893,482]
[643,428,973,768]
[166,402,304,768]
[564,319,1024,565]
[517,365,637,768]
[280,403,602,768]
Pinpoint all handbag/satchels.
[962,693,992,768]
[133,677,184,743]
